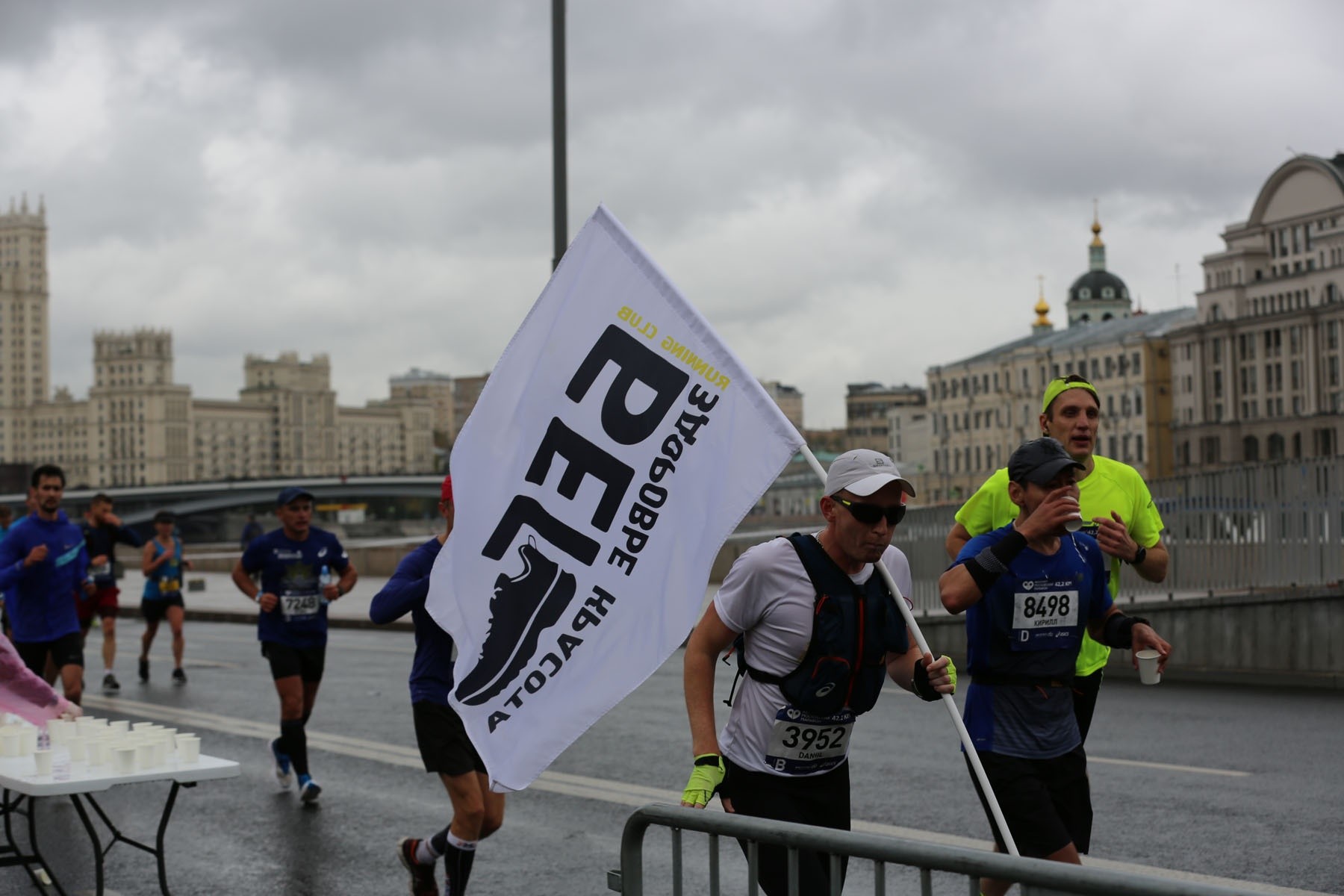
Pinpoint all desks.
[0,751,244,896]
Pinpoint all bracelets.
[255,591,263,602]
[337,584,345,597]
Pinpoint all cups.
[34,749,52,776]
[1060,496,1083,532]
[1135,649,1162,685]
[0,725,23,756]
[46,713,202,774]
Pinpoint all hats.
[276,489,315,508]
[442,474,453,504]
[825,448,916,498]
[1008,438,1087,486]
[1040,375,1099,438]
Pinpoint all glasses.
[829,494,906,526]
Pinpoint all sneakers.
[81,679,85,689]
[298,773,321,801]
[397,836,440,896]
[102,673,121,690]
[270,738,292,788]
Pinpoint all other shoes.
[138,656,149,679]
[172,668,184,678]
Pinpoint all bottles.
[318,565,332,604]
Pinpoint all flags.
[425,204,801,796]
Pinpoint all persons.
[683,449,961,896]
[939,437,1173,896]
[947,376,1170,741]
[0,462,506,895]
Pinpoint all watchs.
[1127,615,1153,637]
[1124,543,1146,568]
